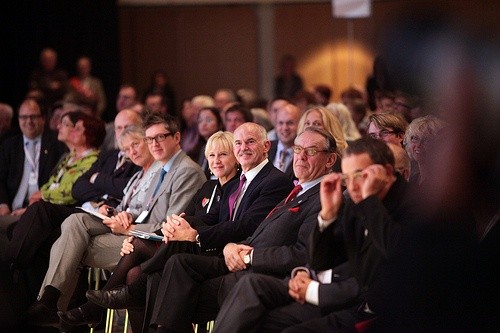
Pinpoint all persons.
[0,49,500,333]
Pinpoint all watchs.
[243,249,252,268]
[195,234,201,244]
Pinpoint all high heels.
[57,302,103,328]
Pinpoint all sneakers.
[27,295,61,328]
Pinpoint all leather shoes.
[85,286,145,311]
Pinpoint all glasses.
[367,130,397,139]
[411,136,435,146]
[291,146,335,156]
[142,131,175,144]
[18,115,43,121]
[340,173,365,186]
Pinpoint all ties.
[279,151,290,169]
[12,140,37,211]
[229,174,247,222]
[266,185,303,219]
[152,169,167,198]
